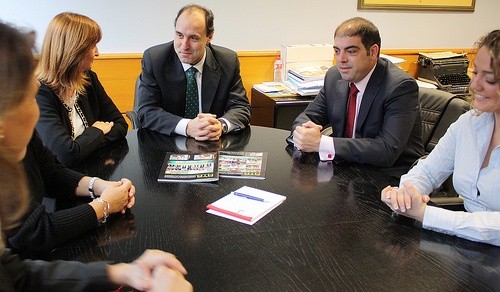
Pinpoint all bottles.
[274,57,284,86]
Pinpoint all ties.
[343,83,358,138]
[184,67,199,119]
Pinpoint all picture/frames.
[357,0,476,12]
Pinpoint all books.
[158,152,218,182]
[285,68,325,96]
[219,151,268,180]
[206,185,286,223]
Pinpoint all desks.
[249,84,315,130]
[44,125,500,292]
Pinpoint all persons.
[4,128,135,259]
[134,4,252,141]
[287,16,425,179]
[0,18,194,291]
[34,12,130,165]
[381,30,500,246]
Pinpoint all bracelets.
[88,177,101,199]
[96,197,112,224]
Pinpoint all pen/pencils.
[232,192,264,202]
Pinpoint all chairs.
[416,85,471,210]
